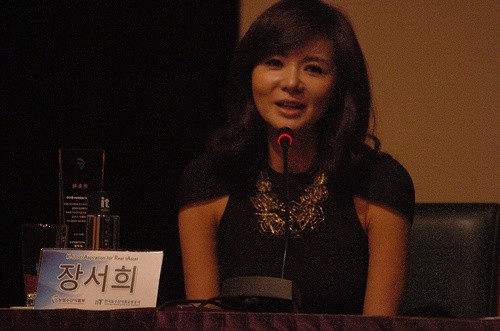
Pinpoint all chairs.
[397,203,500,319]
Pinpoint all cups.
[20,222,69,307]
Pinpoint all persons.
[174,0,415,316]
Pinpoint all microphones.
[220,126,294,306]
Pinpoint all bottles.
[83,190,122,251]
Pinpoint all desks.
[0,307,500,331]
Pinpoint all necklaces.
[249,170,331,241]
